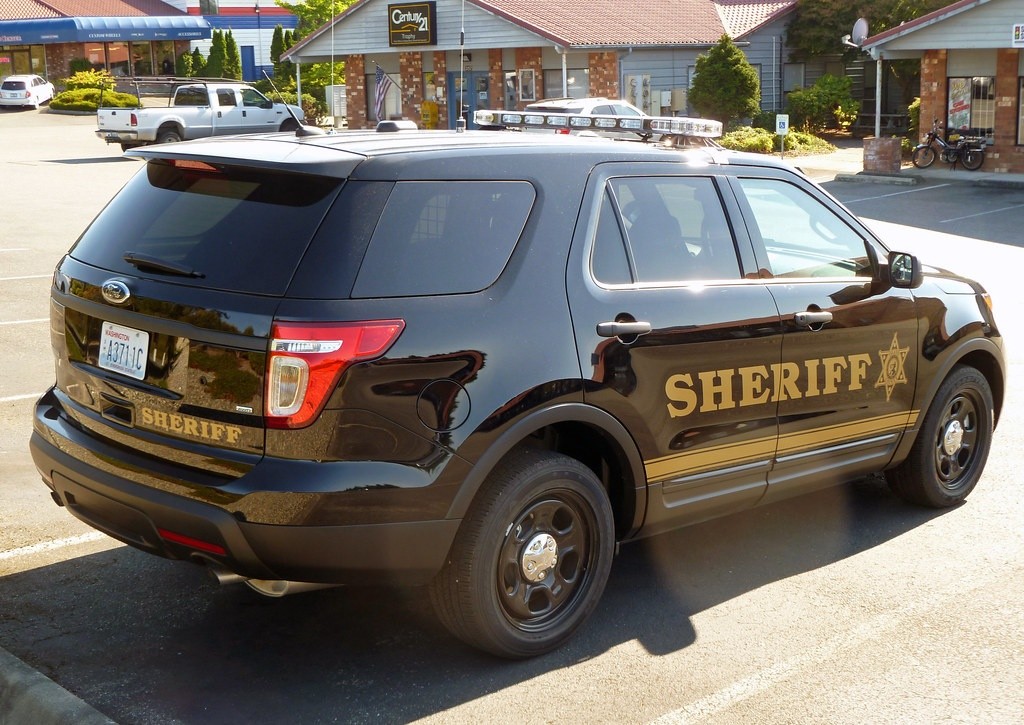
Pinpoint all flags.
[374,65,392,122]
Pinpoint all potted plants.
[901,138,914,158]
[306,109,316,126]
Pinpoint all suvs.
[29,110,1007,658]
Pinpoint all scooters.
[911,115,986,171]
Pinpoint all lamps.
[459,53,471,62]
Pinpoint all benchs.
[849,126,895,131]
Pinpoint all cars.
[0,74,55,110]
[526,97,645,116]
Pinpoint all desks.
[855,114,905,137]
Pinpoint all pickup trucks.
[94,84,308,152]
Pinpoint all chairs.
[627,211,689,282]
[698,210,740,278]
